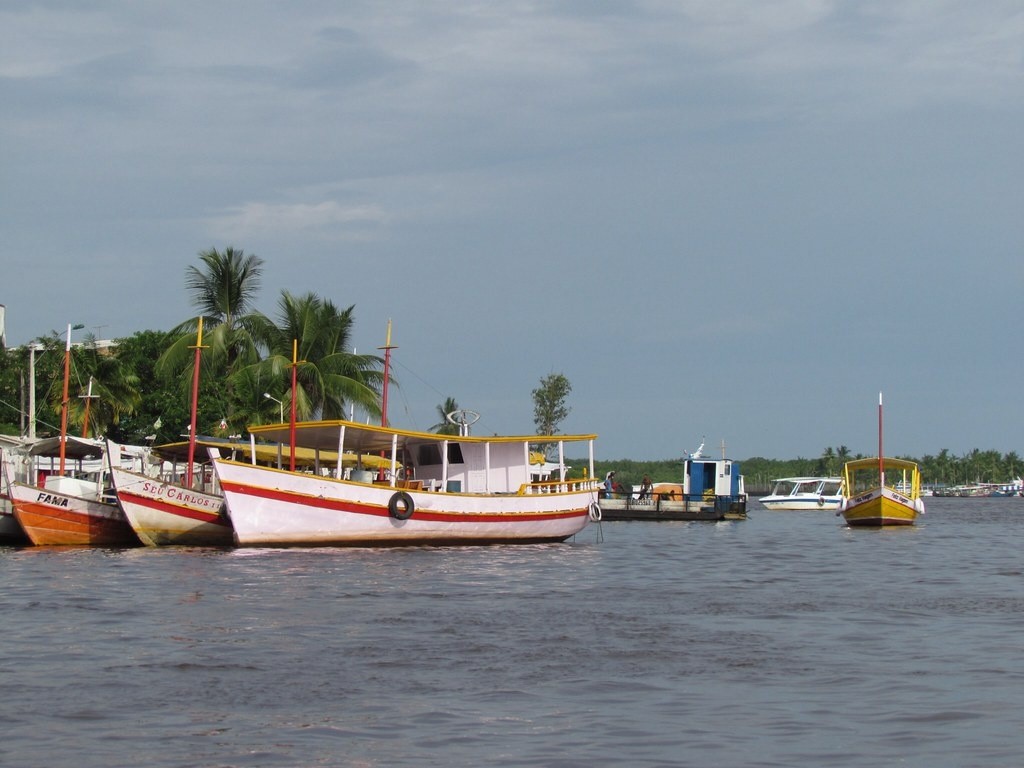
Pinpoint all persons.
[604,472,616,499]
[661,490,676,501]
[641,474,653,489]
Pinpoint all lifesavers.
[387,491,415,520]
[587,498,602,522]
[818,497,826,506]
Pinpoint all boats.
[833,390,924,525]
[636,435,747,519]
[0,322,408,546]
[207,340,600,555]
[758,476,845,511]
[932,479,1023,499]
